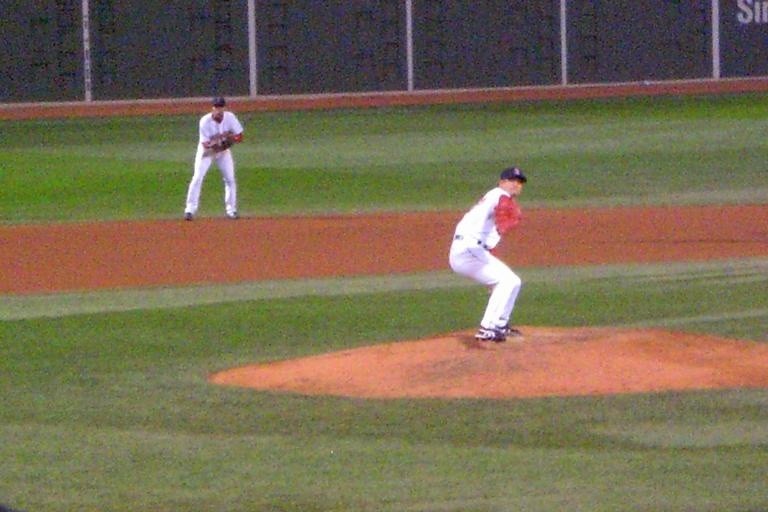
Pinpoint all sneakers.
[229,212,239,220]
[184,213,193,221]
[474,326,506,340]
[496,326,520,336]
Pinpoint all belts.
[454,235,488,247]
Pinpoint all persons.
[181,94,245,222]
[448,166,529,343]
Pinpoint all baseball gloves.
[494,206,520,235]
[210,133,235,152]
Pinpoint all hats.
[502,168,528,183]
[212,97,225,106]
